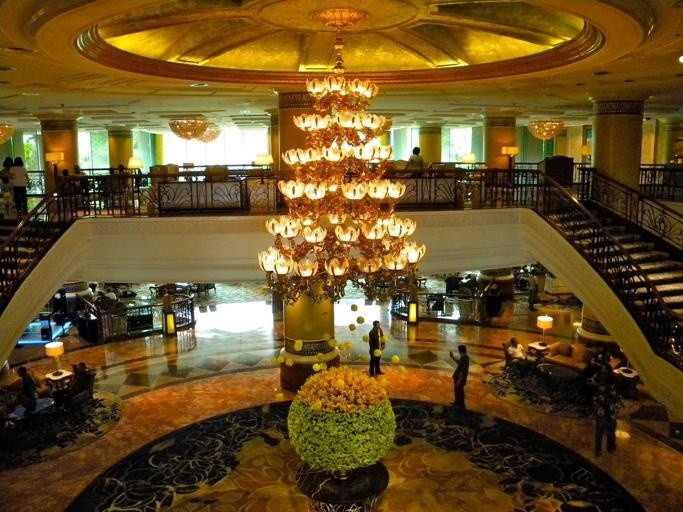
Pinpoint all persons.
[103,288,116,300]
[507,336,538,380]
[448,343,470,410]
[592,371,624,457]
[16,366,37,401]
[93,290,109,306]
[50,361,97,415]
[0,155,129,220]
[527,270,539,310]
[367,320,386,376]
[588,341,616,368]
[402,147,425,178]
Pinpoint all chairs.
[390,264,641,417]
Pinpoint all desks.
[294,459,389,511]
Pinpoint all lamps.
[0,123,14,148]
[43,152,64,189]
[252,151,274,186]
[460,152,476,177]
[194,123,221,144]
[500,144,521,169]
[525,120,565,141]
[255,6,426,308]
[127,155,144,173]
[579,144,592,161]
[167,119,208,141]
